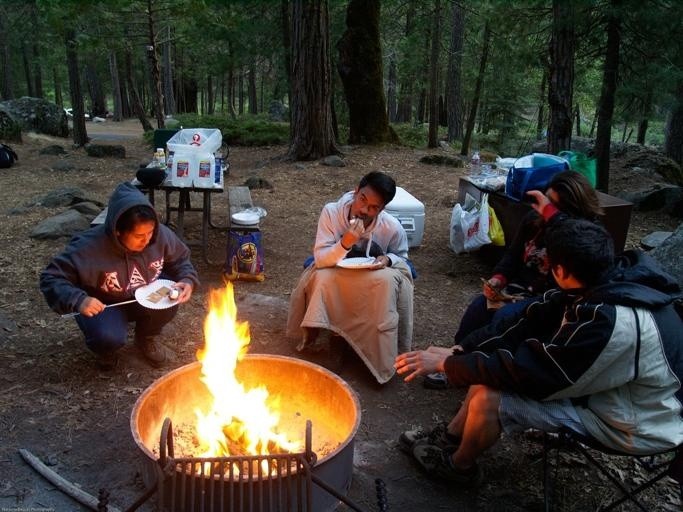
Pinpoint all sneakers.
[96,353,120,368]
[411,439,485,487]
[398,420,461,451]
[133,333,166,369]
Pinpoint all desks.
[457,177,634,271]
[129,168,224,263]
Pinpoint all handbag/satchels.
[221,227,266,282]
[504,151,571,202]
[559,150,598,190]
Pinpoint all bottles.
[470,151,481,178]
[154,147,174,182]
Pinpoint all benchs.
[226,184,260,237]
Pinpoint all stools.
[525,429,681,510]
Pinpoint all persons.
[39,181,198,381]
[313,171,410,366]
[394,220,683,485]
[426,170,600,386]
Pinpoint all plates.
[337,256,381,270]
[232,213,259,225]
[135,280,185,310]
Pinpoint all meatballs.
[169,290,178,299]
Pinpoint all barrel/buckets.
[172,145,215,187]
